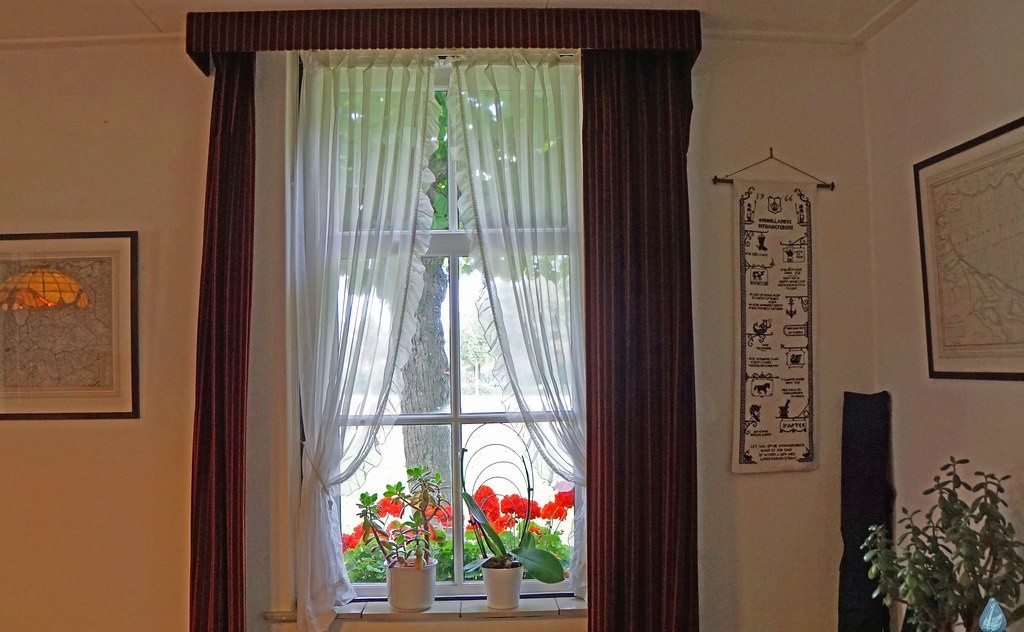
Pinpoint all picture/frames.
[913,116,1023,381]
[0,228,140,419]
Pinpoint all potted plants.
[460,490,565,611]
[357,465,453,612]
